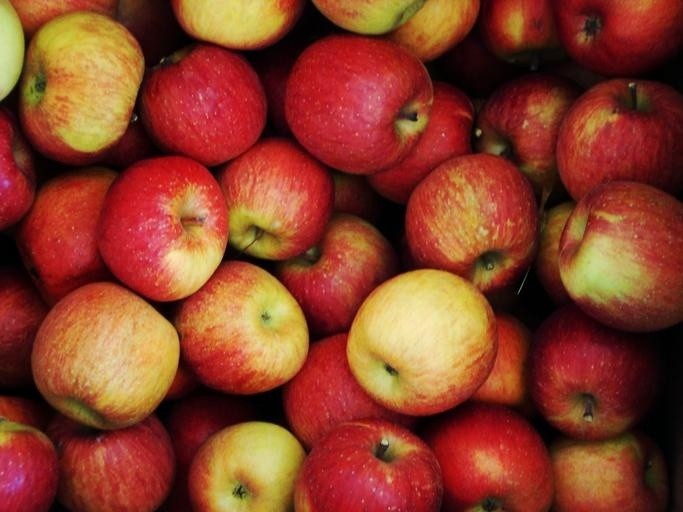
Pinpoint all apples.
[0,0,682,512]
